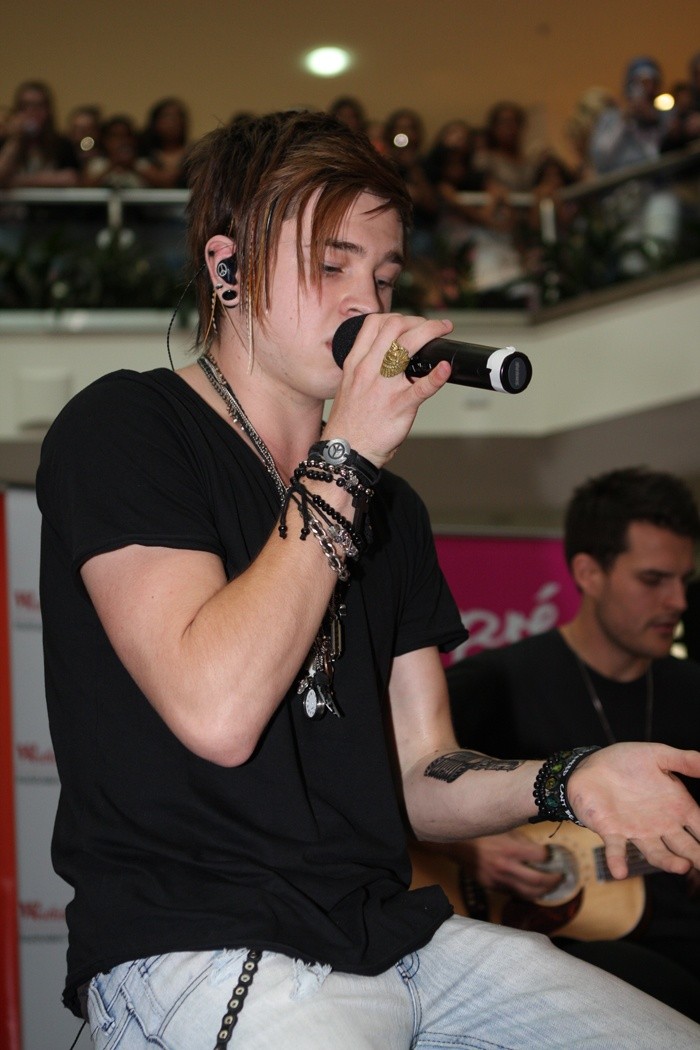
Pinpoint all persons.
[36,108,700,1049]
[0,51,700,312]
[438,467,698,1024]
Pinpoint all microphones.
[330,312,534,396]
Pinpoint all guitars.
[407,818,661,942]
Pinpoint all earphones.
[217,252,239,286]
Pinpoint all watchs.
[307,439,383,490]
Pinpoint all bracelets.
[288,485,358,561]
[533,743,607,828]
[279,491,349,583]
[289,476,362,549]
[293,460,374,505]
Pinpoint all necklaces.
[196,350,345,718]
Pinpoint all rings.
[380,342,410,377]
